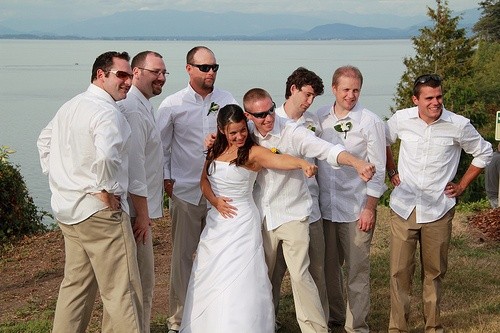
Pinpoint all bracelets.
[388,168,398,179]
[164,182,172,190]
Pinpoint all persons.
[313,66,388,333]
[270,67,331,333]
[180,104,317,333]
[117,51,166,333]
[385,73,493,333]
[204,87,376,333]
[36,52,147,333]
[484,141,500,209]
[155,45,239,333]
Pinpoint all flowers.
[334,121,353,140]
[207,102,219,117]
[272,147,280,154]
[307,123,316,132]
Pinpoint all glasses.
[137,67,170,78]
[187,63,220,73]
[244,101,276,118]
[413,74,443,91]
[100,70,133,82]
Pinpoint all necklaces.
[221,144,239,156]
